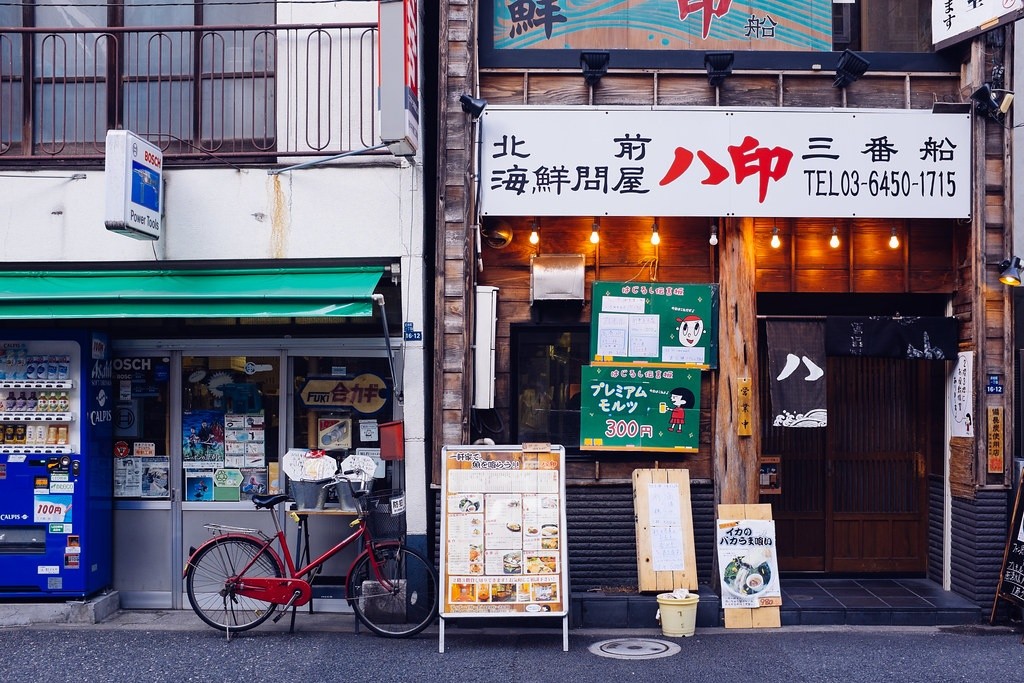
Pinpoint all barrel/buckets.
[655,593,700,638]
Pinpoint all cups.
[745,574,762,592]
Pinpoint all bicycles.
[182,470,439,644]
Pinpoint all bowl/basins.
[724,557,776,599]
[468,506,475,511]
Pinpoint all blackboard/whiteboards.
[995,466,1024,611]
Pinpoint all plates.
[507,523,522,532]
[526,605,541,612]
[458,497,480,512]
[542,524,558,534]
[525,531,540,537]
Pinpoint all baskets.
[360,488,407,540]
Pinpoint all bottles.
[59,391,69,412]
[0,351,70,381]
[28,392,38,411]
[38,391,48,411]
[6,391,16,411]
[49,392,58,412]
[0,421,67,447]
[17,392,27,412]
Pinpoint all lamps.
[830,218,840,248]
[704,51,734,86]
[889,218,899,249]
[650,224,660,245]
[459,93,489,120]
[527,222,539,244]
[969,83,1000,113]
[590,223,601,243]
[771,216,781,248]
[708,216,719,245]
[998,257,1022,286]
[831,49,870,89]
[578,51,612,87]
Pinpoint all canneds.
[0,424,67,442]
[25,360,71,381]
